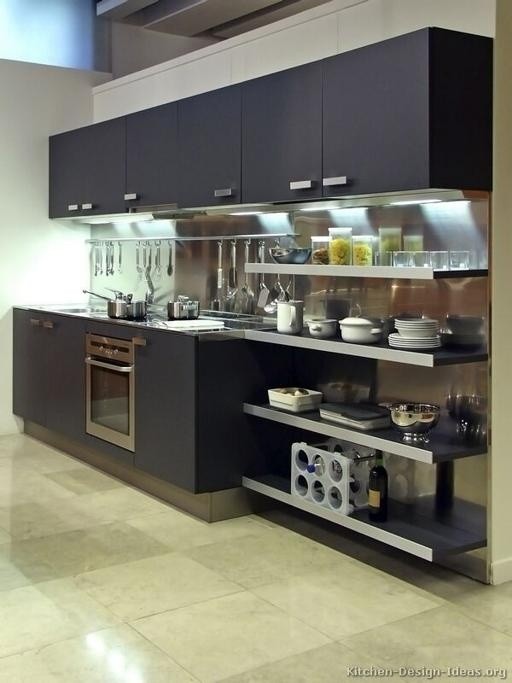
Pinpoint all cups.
[277,300,303,333]
[414,250,469,270]
[326,298,361,321]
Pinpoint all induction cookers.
[89,310,208,324]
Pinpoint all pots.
[307,315,384,344]
[168,295,200,319]
[82,288,147,320]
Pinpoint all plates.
[387,318,441,350]
[438,313,484,352]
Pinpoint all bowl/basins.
[269,249,313,264]
[387,403,440,438]
[446,395,489,426]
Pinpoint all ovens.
[83,334,136,452]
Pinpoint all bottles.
[311,228,424,267]
[308,457,342,479]
[368,448,388,523]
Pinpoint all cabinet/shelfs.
[14,310,85,463]
[242,27,493,204]
[239,262,490,564]
[48,116,126,219]
[134,328,293,524]
[127,83,241,211]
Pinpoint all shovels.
[257,241,270,307]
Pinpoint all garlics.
[280,388,308,396]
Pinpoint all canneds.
[310,227,424,266]
